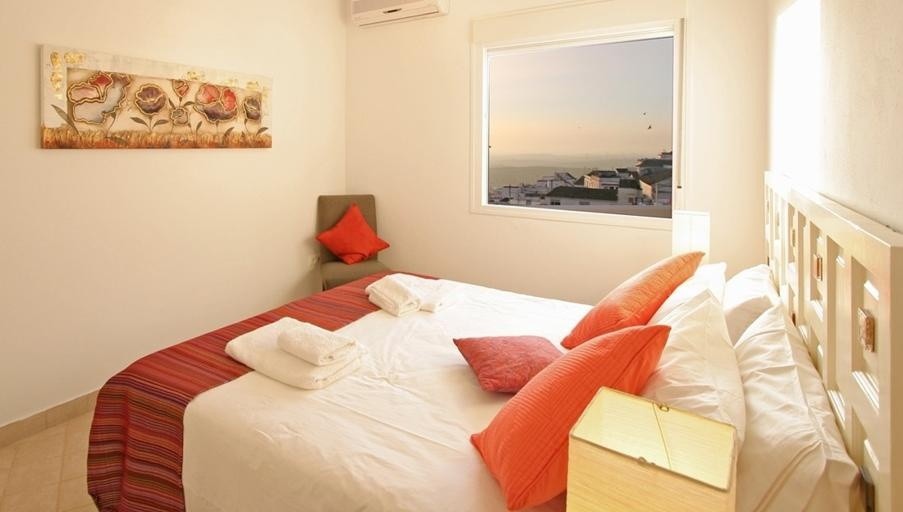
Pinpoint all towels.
[364,271,446,317]
[222,316,368,392]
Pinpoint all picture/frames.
[481,32,679,217]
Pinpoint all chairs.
[317,194,387,291]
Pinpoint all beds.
[87,169,903,512]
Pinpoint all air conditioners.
[350,0,450,29]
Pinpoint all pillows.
[316,202,390,264]
